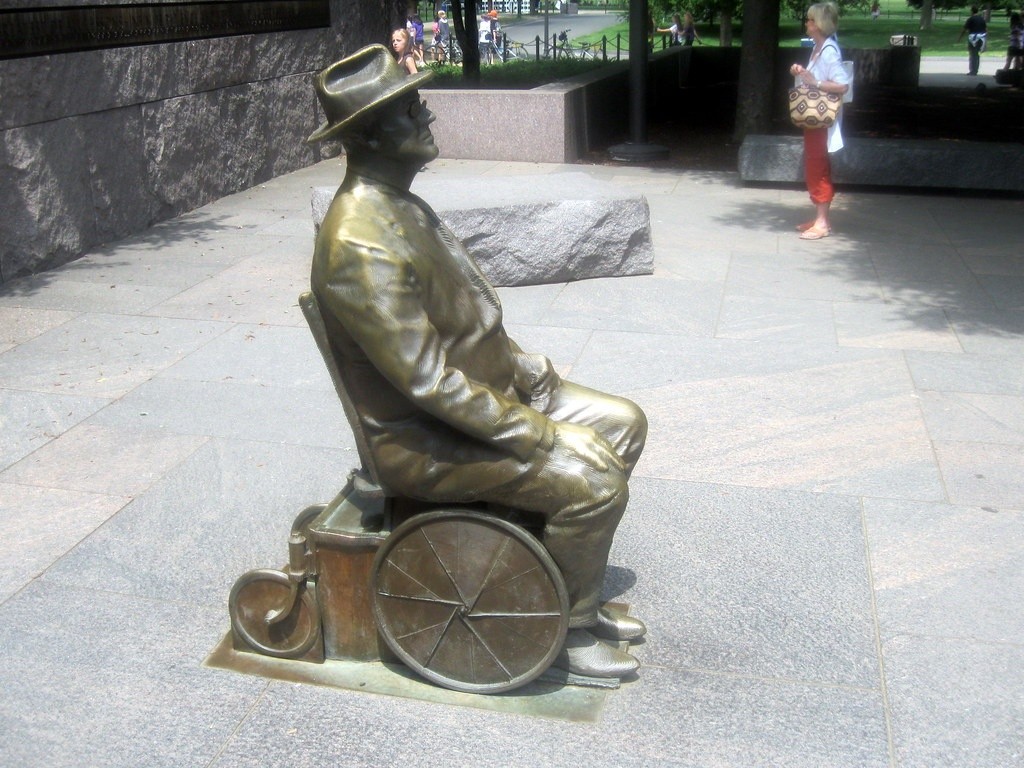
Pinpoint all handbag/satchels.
[435,31,441,40]
[786,45,843,130]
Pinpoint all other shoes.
[967,72,977,76]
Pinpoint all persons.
[791,3,849,239]
[392,28,418,75]
[431,15,441,61]
[413,14,423,62]
[478,14,490,59]
[657,14,683,47]
[306,45,645,677]
[958,6,986,74]
[1004,8,1024,69]
[436,10,449,41]
[488,10,502,64]
[678,14,702,45]
[407,15,414,51]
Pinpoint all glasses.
[804,15,815,24]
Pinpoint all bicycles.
[545,28,605,61]
[479,31,528,65]
[423,33,464,68]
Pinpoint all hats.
[488,10,498,17]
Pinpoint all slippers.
[798,226,830,240]
[795,220,832,232]
